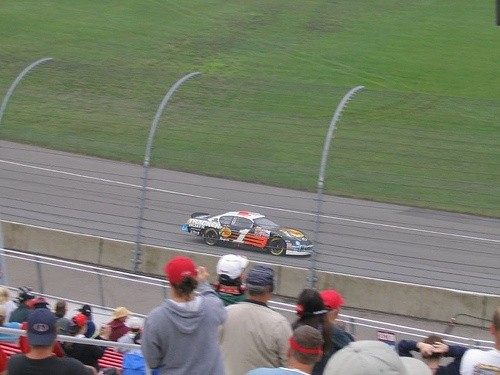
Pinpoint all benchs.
[0,322,125,374]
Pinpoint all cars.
[181,210,314,257]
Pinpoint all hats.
[320,290,345,309]
[56,318,74,332]
[32,297,50,306]
[111,306,133,319]
[289,325,324,364]
[322,340,433,375]
[216,254,249,280]
[27,307,57,346]
[78,304,91,314]
[296,288,327,316]
[19,293,35,303]
[72,314,86,325]
[246,264,276,292]
[165,255,196,287]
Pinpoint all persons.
[245,288,500,375]
[0,286,160,375]
[140,253,293,375]
